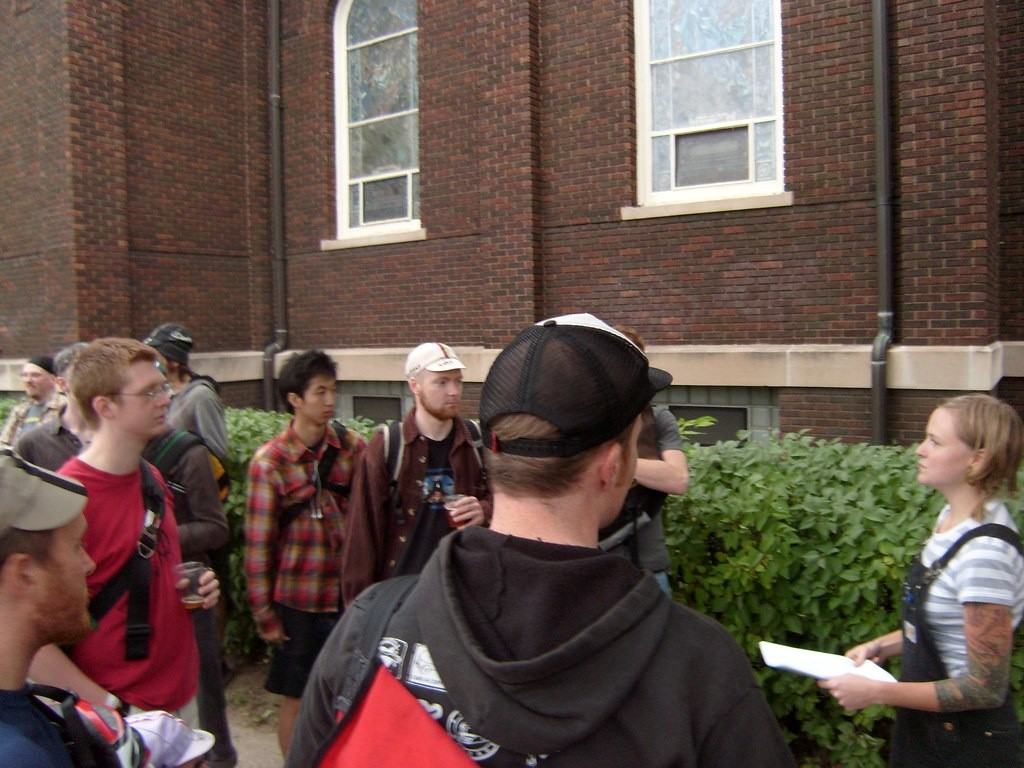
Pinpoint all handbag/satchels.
[151,429,230,506]
[295,571,483,768]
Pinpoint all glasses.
[107,381,175,400]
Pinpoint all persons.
[0,321,689,768]
[816,391,1024,768]
[282,310,798,768]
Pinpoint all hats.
[123,710,216,768]
[404,342,467,378]
[0,444,89,534]
[29,354,58,377]
[478,311,674,458]
[142,322,194,365]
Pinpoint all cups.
[171,561,205,610]
[442,493,467,527]
